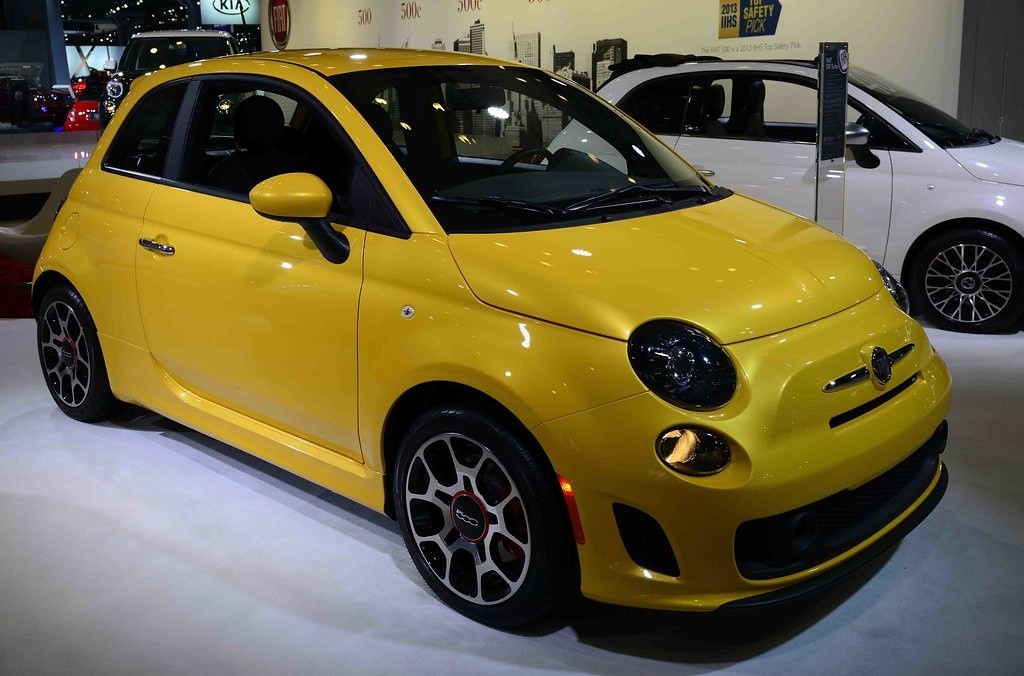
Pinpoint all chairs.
[204,93,425,220]
[148,46,177,69]
[700,79,772,140]
[0,167,84,293]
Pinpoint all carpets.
[0,251,36,319]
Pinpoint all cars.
[542,56,1024,333]
[32,48,953,629]
[0,31,252,143]
